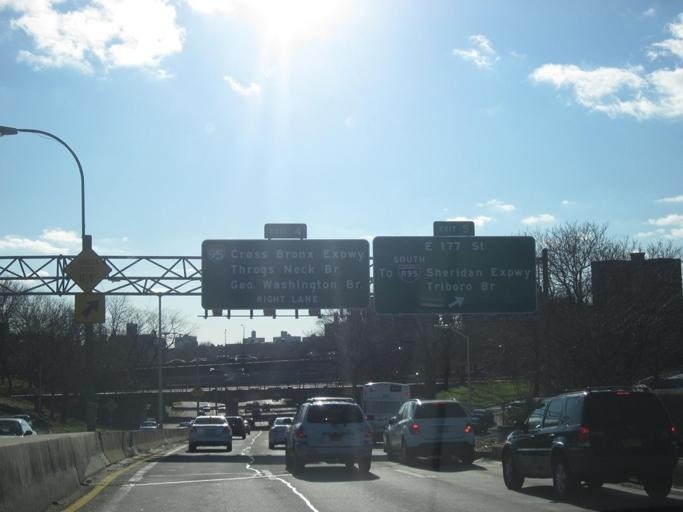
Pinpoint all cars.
[0,417,38,438]
[136,420,160,430]
[179,400,295,452]
[161,353,258,367]
[10,414,33,428]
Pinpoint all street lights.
[0,126,98,433]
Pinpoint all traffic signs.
[373,235,541,317]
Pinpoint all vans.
[359,379,411,445]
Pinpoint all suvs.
[382,397,477,464]
[501,383,680,500]
[284,396,375,474]
